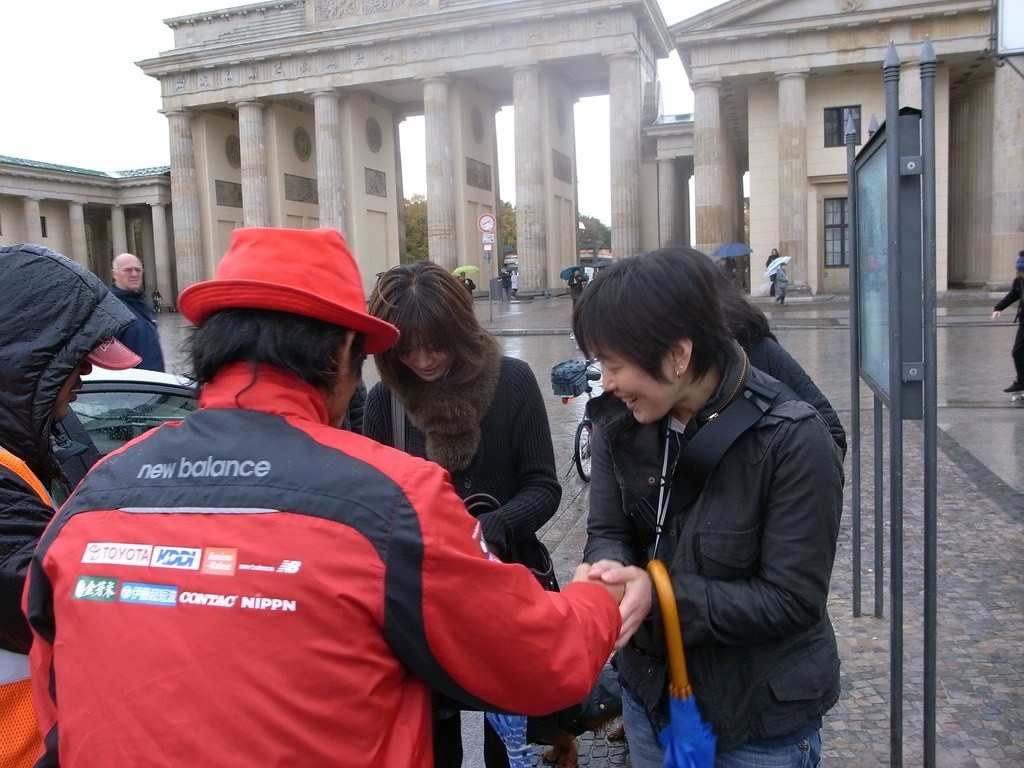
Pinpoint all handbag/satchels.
[519,543,562,594]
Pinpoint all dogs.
[526,667,626,768]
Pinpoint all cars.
[49,364,201,504]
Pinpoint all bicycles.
[552,334,605,481]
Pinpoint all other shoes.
[774,299,780,306]
[779,304,786,308]
[1003,381,1024,393]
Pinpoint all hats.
[179,224,400,355]
[1014,256,1024,271]
[84,336,143,373]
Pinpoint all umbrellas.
[452,265,479,285]
[712,243,753,258]
[764,257,791,279]
[591,261,612,268]
[560,265,585,282]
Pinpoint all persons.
[498,269,518,302]
[991,251,1024,392]
[775,263,788,303]
[0,244,142,768]
[363,261,559,595]
[725,256,748,289]
[568,272,583,340]
[460,279,475,301]
[576,248,846,768]
[765,249,780,296]
[109,253,165,374]
[151,289,162,310]
[23,232,626,768]
